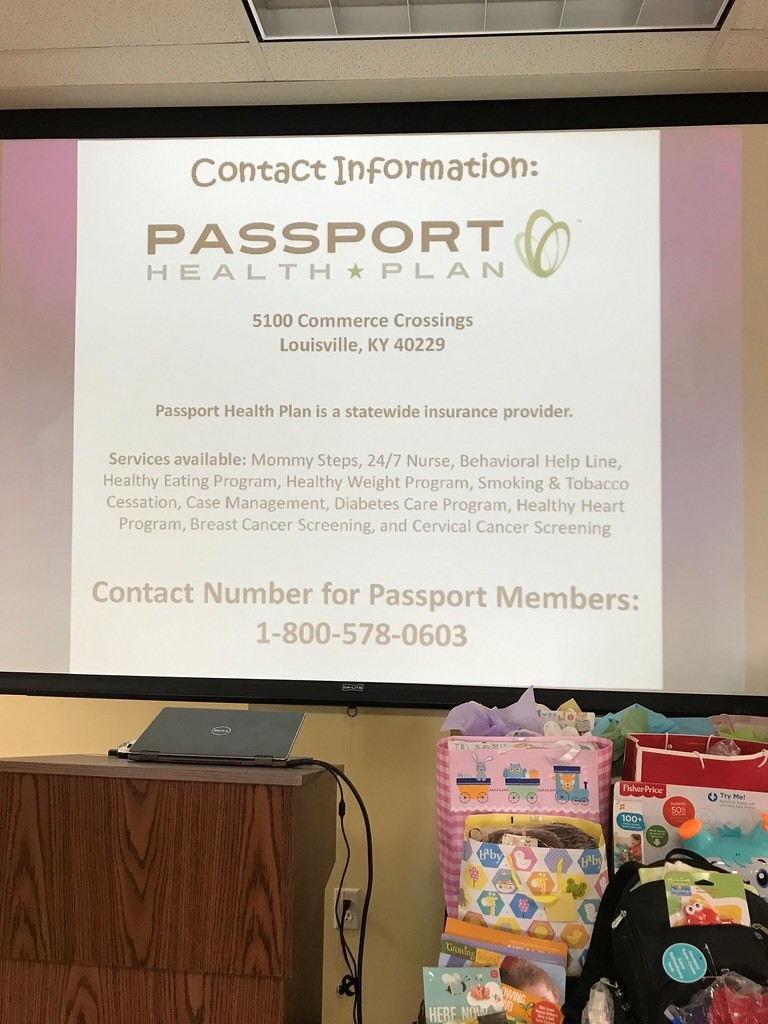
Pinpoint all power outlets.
[334,889,362,931]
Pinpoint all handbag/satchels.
[437,714,768,1024]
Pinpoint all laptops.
[129,707,314,767]
